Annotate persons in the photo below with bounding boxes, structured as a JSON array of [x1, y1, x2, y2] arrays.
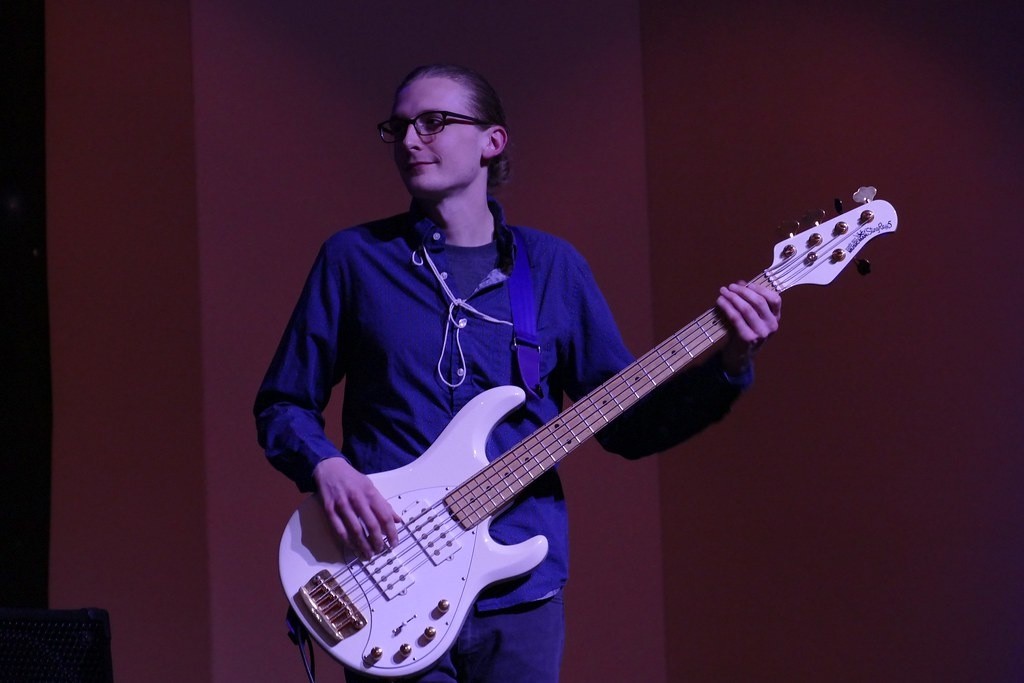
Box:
[[250, 62, 788, 683]]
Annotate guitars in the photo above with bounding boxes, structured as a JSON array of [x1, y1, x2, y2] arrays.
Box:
[[278, 186, 899, 676]]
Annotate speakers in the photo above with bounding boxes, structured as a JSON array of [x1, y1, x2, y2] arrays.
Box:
[[0, 608, 115, 683]]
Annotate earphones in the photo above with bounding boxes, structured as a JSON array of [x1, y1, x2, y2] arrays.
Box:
[[459, 318, 467, 328]]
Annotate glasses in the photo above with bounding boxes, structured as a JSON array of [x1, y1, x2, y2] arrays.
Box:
[[376, 110, 497, 143]]
